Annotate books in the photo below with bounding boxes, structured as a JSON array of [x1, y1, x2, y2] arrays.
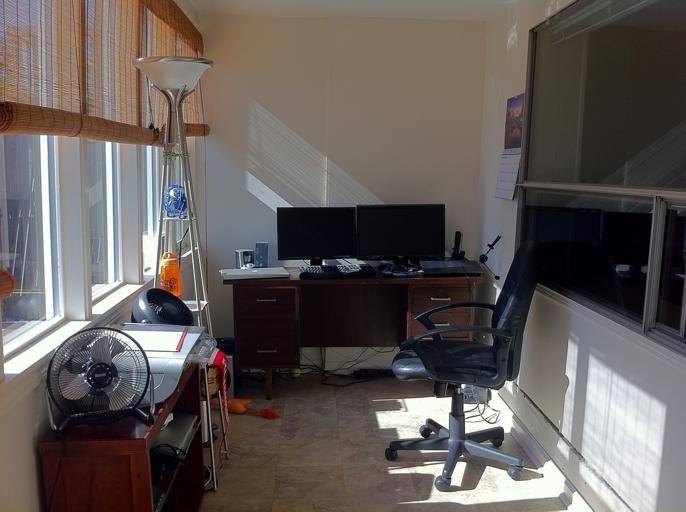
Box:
[[421, 257, 483, 277], [220, 266, 290, 280]]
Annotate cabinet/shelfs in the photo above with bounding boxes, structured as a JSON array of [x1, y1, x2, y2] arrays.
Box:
[[129, 55, 213, 335], [232, 283, 301, 400], [40, 365, 203, 511], [398, 286, 475, 346]]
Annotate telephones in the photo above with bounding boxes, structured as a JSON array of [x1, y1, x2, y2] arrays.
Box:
[[451, 230, 465, 260]]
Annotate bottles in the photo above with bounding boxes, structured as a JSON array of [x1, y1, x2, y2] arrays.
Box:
[[160, 252, 183, 302]]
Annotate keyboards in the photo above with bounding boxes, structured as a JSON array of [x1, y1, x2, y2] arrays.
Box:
[[296, 265, 376, 279]]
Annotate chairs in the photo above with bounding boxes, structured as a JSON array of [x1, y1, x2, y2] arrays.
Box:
[[384, 232, 550, 491]]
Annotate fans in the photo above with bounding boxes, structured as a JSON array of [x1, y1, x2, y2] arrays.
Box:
[[46, 326, 156, 434]]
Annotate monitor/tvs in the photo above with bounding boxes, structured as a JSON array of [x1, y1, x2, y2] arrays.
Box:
[[356, 203, 445, 271], [277, 207, 355, 267]]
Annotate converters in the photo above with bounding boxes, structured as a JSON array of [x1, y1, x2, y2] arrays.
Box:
[[357, 369, 378, 378], [151, 450, 176, 476]]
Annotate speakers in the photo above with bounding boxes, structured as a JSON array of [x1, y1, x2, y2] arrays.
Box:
[[256, 242, 268, 267]]
